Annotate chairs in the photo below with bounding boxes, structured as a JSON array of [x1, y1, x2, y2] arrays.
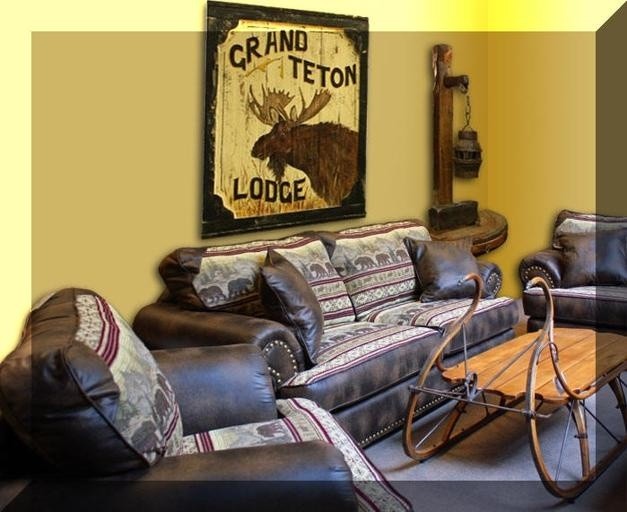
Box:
[[518, 211, 627, 333], [0, 287, 411, 511]]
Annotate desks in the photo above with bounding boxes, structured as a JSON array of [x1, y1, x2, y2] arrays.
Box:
[[403, 272, 626, 500]]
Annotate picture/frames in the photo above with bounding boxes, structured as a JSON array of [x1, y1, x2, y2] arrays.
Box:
[[201, 0, 369, 239]]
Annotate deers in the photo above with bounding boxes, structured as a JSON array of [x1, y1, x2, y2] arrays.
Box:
[[247, 84, 358, 208]]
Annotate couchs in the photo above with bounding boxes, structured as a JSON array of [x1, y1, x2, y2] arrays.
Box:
[[132, 287, 519, 450]]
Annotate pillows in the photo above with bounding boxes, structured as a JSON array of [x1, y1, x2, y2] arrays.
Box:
[[159, 233, 356, 324], [259, 245, 324, 366], [405, 238, 491, 301], [554, 218, 627, 289], [320, 220, 431, 318]]
